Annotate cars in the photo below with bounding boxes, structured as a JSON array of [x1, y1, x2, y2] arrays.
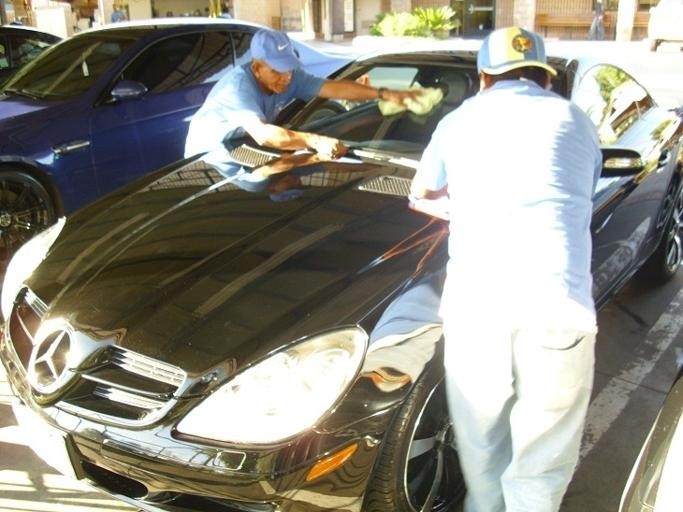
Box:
[[648, 0, 683, 52]]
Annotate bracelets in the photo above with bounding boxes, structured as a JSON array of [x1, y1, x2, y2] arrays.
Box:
[[378, 88, 388, 99]]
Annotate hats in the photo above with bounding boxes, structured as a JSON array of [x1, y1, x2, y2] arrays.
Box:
[[476, 24, 559, 78], [249, 28, 308, 74]]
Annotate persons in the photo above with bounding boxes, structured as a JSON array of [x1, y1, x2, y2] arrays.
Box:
[[181, 28, 423, 159], [408, 27, 601, 510], [110, 3, 126, 25], [197, 144, 324, 202], [167, 2, 234, 22], [582, 1, 606, 41]]
